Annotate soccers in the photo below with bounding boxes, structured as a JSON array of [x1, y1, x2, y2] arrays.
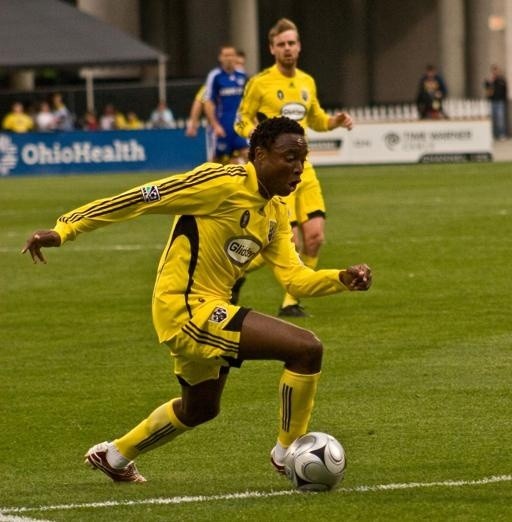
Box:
[[285, 431, 346, 490]]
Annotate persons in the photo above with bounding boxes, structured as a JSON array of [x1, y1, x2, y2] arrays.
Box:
[[181, 48, 250, 164], [483, 62, 510, 141], [21, 113, 373, 481], [230, 17, 354, 318], [416, 63, 452, 118], [2, 92, 177, 133]]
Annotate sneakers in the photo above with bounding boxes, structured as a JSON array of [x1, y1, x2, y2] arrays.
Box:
[[270, 446, 286, 475], [277, 301, 308, 317], [84, 440, 148, 483]]
